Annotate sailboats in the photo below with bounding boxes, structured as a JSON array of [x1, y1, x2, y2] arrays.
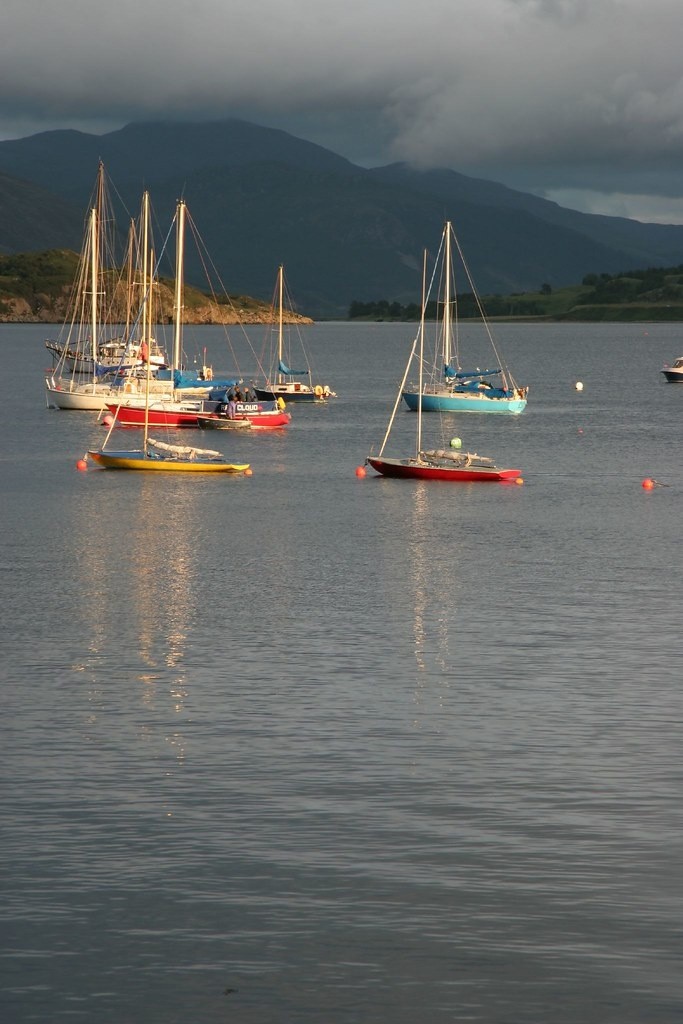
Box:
[[86, 250, 249, 473], [400, 221, 529, 415], [44, 159, 292, 429], [250, 267, 323, 400], [366, 248, 521, 481]]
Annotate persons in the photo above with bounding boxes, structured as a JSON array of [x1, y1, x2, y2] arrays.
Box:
[[226, 397, 238, 420], [243, 386, 258, 402], [234, 387, 246, 403]]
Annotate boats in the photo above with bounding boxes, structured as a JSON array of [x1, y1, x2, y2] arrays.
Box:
[[661, 357, 683, 383]]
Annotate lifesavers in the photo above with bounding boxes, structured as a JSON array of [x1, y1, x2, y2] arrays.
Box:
[[315, 385, 323, 396], [278, 397, 286, 409]]
